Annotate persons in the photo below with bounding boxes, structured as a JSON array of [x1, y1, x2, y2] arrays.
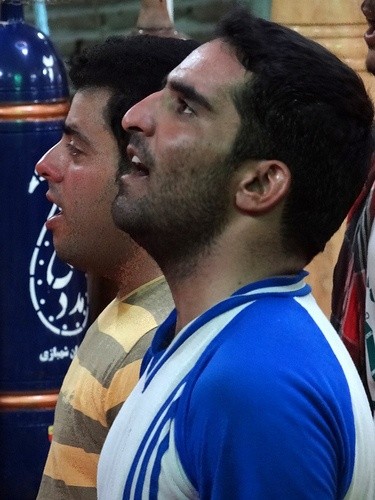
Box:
[[329, 1, 375, 425], [35, 36, 212, 500], [95, 10, 374, 500]]
